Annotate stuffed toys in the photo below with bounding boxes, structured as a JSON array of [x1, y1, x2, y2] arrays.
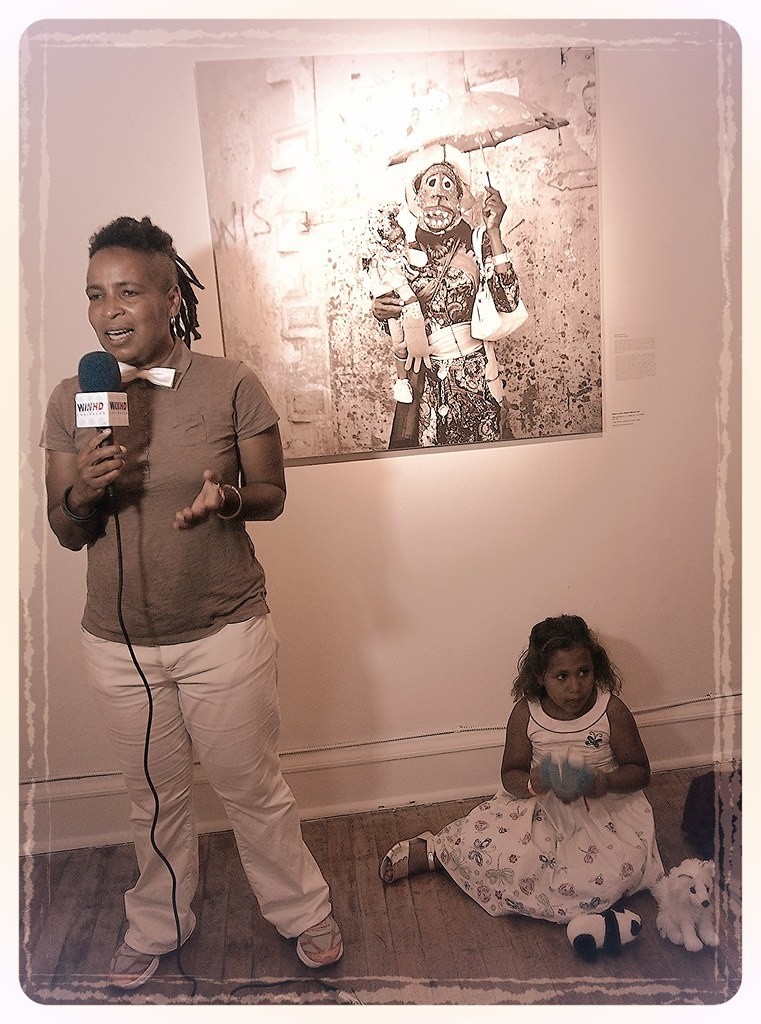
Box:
[[653, 858, 720, 952], [566, 906, 643, 962]]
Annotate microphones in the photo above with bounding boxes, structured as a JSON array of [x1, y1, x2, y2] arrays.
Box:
[[74, 350, 129, 499]]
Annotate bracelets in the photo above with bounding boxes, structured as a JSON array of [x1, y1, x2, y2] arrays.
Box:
[[60, 485, 97, 522], [492, 252, 511, 266], [211, 482, 243, 520], [528, 778, 540, 797]]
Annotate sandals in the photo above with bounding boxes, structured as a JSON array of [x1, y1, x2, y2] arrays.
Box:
[[378, 830, 436, 884]]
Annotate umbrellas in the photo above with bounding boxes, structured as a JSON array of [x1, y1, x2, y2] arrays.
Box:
[[385, 90, 570, 185]]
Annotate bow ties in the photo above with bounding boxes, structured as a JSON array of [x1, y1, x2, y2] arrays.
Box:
[[117, 361, 175, 388]]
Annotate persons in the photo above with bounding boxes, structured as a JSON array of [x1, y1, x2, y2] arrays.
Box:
[[368, 162, 528, 450], [40, 215, 344, 990], [379, 613, 664, 953]]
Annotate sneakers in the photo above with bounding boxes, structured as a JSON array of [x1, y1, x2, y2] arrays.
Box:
[[296, 914, 343, 968], [109, 942, 159, 988]]
